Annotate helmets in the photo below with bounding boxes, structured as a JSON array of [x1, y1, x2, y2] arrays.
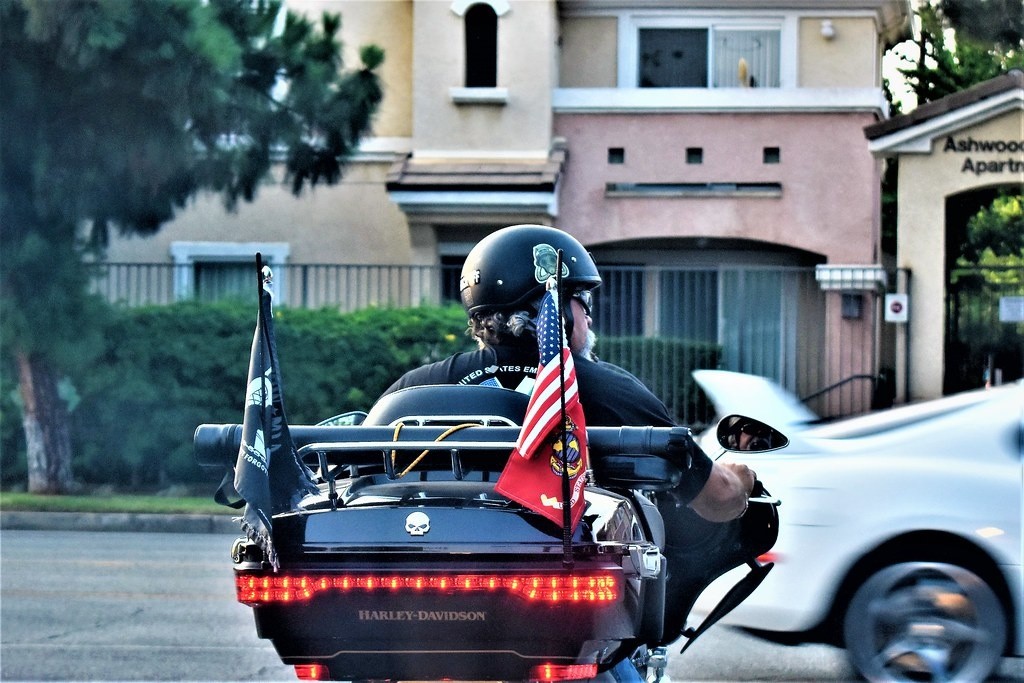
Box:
[[729, 416, 743, 433], [457, 223, 603, 325]]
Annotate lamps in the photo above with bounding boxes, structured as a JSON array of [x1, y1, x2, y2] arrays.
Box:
[[820, 21, 835, 40]]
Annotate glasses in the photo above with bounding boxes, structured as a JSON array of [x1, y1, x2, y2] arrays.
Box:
[[569, 287, 594, 318], [739, 422, 774, 435]]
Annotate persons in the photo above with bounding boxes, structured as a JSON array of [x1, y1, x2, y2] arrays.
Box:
[[358, 222, 756, 683], [727, 415, 772, 450]]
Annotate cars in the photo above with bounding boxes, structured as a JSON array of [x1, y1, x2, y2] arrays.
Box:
[[676, 368, 1024, 682]]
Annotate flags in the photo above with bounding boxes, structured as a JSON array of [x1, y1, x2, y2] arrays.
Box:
[[494, 275, 593, 536], [232, 285, 320, 572]]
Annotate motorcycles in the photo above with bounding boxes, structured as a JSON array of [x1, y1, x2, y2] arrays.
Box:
[[195, 248, 792, 683]]
